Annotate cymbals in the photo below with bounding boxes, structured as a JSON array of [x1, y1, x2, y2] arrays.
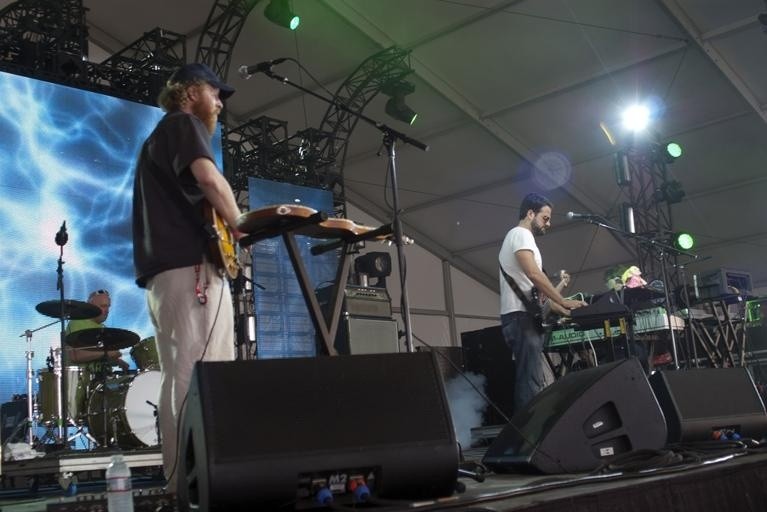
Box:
[[36, 299, 101, 320], [65, 328, 140, 352]]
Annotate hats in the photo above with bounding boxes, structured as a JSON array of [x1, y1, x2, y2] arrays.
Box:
[[167, 63, 234, 100]]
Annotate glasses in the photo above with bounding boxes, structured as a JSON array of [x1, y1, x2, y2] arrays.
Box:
[[89, 289, 109, 301]]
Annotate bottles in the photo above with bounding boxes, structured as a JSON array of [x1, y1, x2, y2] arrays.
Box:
[[105, 454, 133, 511], [67, 433, 75, 449]]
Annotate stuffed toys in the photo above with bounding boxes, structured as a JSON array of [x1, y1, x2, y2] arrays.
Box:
[[619, 265, 647, 289]]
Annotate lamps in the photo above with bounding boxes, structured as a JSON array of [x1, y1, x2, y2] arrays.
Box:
[[383, 92, 417, 126], [262, 1, 300, 32]]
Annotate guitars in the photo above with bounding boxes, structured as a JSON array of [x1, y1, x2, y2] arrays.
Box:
[[203, 198, 243, 279], [532, 269, 571, 336], [236, 203, 414, 245]]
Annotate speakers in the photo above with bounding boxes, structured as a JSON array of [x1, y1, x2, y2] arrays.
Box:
[[0, 400, 35, 445], [416, 346, 470, 385], [461, 324, 515, 427], [176, 351, 460, 512], [647, 367, 767, 445], [481, 356, 668, 477]]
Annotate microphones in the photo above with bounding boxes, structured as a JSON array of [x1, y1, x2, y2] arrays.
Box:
[[238, 57, 283, 80], [567, 212, 600, 221], [55, 221, 68, 245]]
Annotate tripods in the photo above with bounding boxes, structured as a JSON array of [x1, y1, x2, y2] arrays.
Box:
[[32, 270, 89, 455], [32, 365, 100, 449], [4, 334, 58, 446]]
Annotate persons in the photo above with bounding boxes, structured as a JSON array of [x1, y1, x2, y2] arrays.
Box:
[[62, 289, 131, 419], [541, 268, 572, 318], [495, 191, 591, 416], [132, 62, 252, 498]]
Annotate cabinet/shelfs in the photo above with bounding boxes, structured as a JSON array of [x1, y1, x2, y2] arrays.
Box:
[[231, 203, 401, 357], [686, 290, 759, 369]]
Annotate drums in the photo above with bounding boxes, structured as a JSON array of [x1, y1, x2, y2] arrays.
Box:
[[130, 336, 161, 374], [88, 368, 161, 451], [36, 365, 93, 428]]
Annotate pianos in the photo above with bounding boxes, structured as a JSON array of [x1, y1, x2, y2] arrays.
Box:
[[571, 287, 633, 331]]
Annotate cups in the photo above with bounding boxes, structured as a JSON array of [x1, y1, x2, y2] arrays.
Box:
[[745, 300, 760, 322]]
